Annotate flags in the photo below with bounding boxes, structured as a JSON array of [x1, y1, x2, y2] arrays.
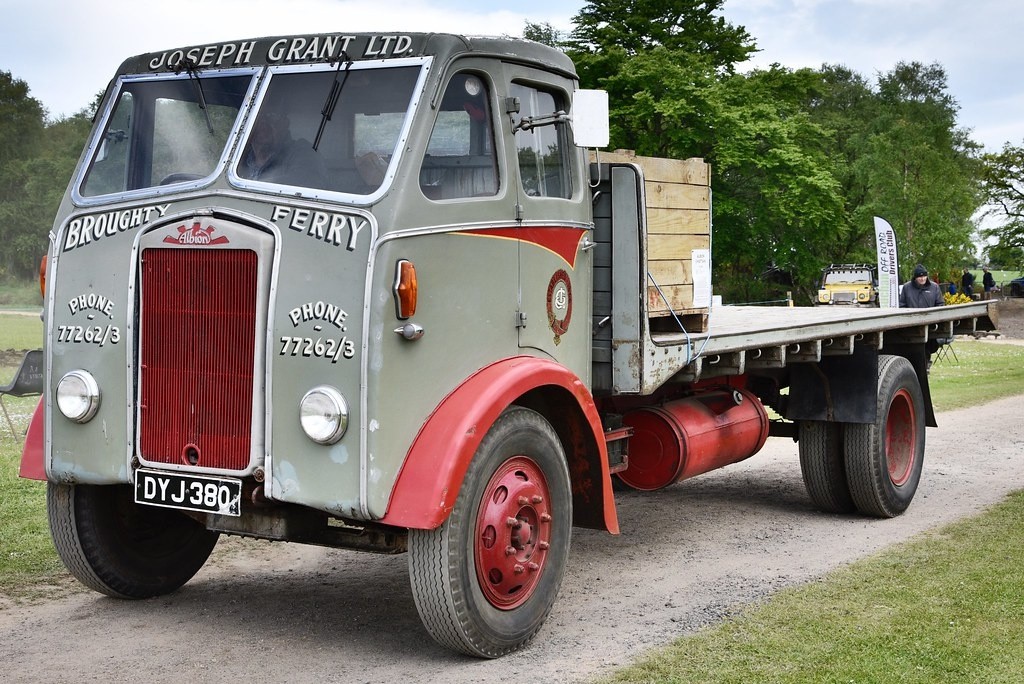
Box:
[[872, 215, 900, 310]]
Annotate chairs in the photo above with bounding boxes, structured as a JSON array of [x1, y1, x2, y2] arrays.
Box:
[[0, 349, 44, 446], [933, 337, 960, 366]]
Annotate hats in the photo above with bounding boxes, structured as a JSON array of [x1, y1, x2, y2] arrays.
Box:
[[914, 264, 928, 280]]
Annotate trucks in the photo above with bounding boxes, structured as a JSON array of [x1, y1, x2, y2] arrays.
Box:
[[814, 262, 880, 308], [16, 31, 1002, 665]]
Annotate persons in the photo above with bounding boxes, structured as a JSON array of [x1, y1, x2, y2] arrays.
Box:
[[982, 265, 994, 292], [898, 263, 947, 307], [950, 281, 957, 294], [238, 94, 328, 195], [960, 266, 976, 299]]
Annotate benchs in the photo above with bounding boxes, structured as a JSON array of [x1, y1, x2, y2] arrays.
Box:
[[323, 155, 499, 199]]
